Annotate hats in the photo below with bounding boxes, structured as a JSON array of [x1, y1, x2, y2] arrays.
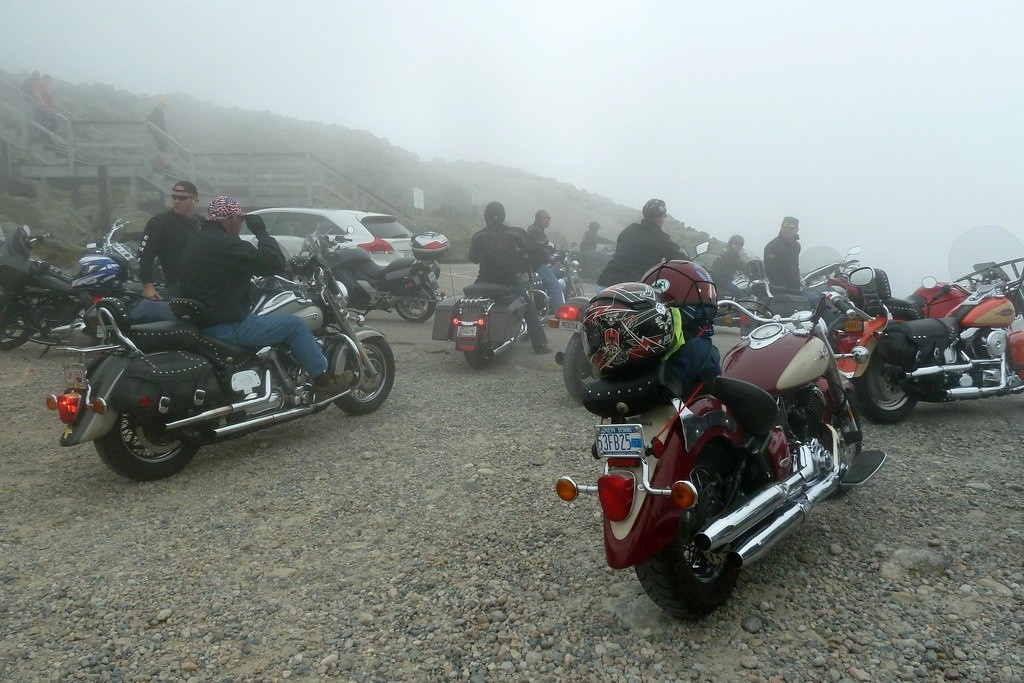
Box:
[[173, 181, 198, 196], [205, 196, 246, 221], [643, 199, 666, 217]]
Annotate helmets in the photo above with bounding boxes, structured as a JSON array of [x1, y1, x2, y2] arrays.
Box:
[[641, 260, 717, 322], [582, 282, 674, 376]]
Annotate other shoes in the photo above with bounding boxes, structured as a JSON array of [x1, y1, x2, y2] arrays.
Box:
[[535, 347, 553, 354]]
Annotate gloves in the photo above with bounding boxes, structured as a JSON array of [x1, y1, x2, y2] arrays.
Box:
[[244, 215, 266, 234]]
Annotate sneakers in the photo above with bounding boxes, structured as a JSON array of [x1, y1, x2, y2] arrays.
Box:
[[313, 371, 353, 396]]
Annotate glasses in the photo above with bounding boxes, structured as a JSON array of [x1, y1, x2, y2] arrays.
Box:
[[172, 195, 192, 201], [781, 225, 798, 231]]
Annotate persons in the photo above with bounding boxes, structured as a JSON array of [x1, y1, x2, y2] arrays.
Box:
[[528, 210, 563, 315], [180, 196, 353, 401], [712, 235, 746, 299], [764, 216, 801, 296], [137, 181, 206, 300], [597, 199, 693, 295], [580, 222, 614, 262], [468, 201, 553, 355]]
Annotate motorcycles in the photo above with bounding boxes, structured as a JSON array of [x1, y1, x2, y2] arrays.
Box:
[[529, 244, 587, 325], [291, 219, 450, 323], [430, 251, 550, 370], [78, 216, 167, 284], [0, 217, 127, 350], [554, 249, 888, 622], [47, 220, 393, 481], [690, 243, 987, 399], [836, 258, 1023, 424]]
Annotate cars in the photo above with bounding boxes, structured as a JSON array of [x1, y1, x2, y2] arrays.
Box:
[[236, 207, 414, 277]]
